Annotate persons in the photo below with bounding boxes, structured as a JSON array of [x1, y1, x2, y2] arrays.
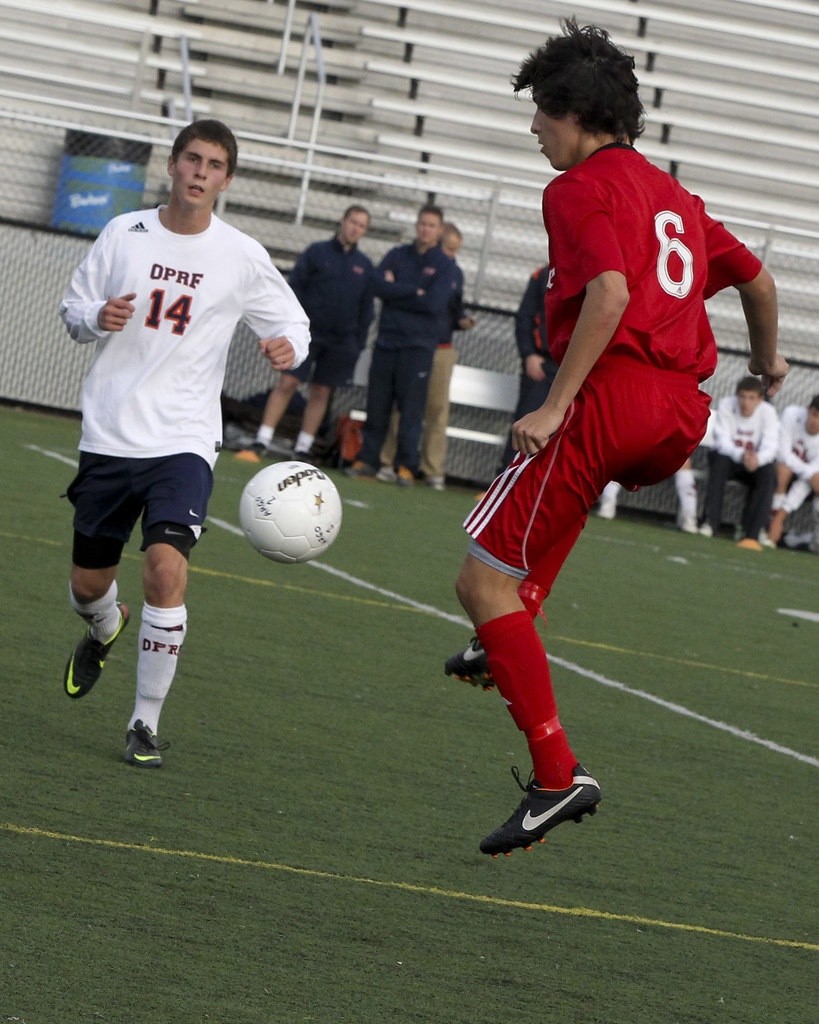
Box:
[[235, 202, 478, 492], [53, 120, 315, 766], [592, 370, 819, 552], [430, 21, 792, 861]]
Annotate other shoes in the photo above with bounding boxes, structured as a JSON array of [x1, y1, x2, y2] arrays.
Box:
[[599, 496, 617, 520], [350, 460, 377, 479], [236, 442, 266, 464], [699, 521, 714, 537], [425, 475, 446, 491], [396, 464, 415, 486], [734, 524, 747, 544], [756, 526, 778, 550], [680, 516, 699, 534], [290, 447, 313, 466], [375, 467, 398, 483]]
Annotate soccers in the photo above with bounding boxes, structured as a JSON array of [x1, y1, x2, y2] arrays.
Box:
[[237, 459, 345, 566]]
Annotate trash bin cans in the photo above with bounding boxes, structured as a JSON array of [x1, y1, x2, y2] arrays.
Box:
[[47, 125, 156, 243]]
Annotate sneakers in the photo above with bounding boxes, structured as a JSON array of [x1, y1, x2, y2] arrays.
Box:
[[123, 719, 172, 768], [445, 636, 496, 691], [63, 601, 130, 698], [479, 762, 601, 858]]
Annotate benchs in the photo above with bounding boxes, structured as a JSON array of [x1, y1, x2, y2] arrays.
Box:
[[0, 1, 817, 364], [348, 348, 519, 445]]
